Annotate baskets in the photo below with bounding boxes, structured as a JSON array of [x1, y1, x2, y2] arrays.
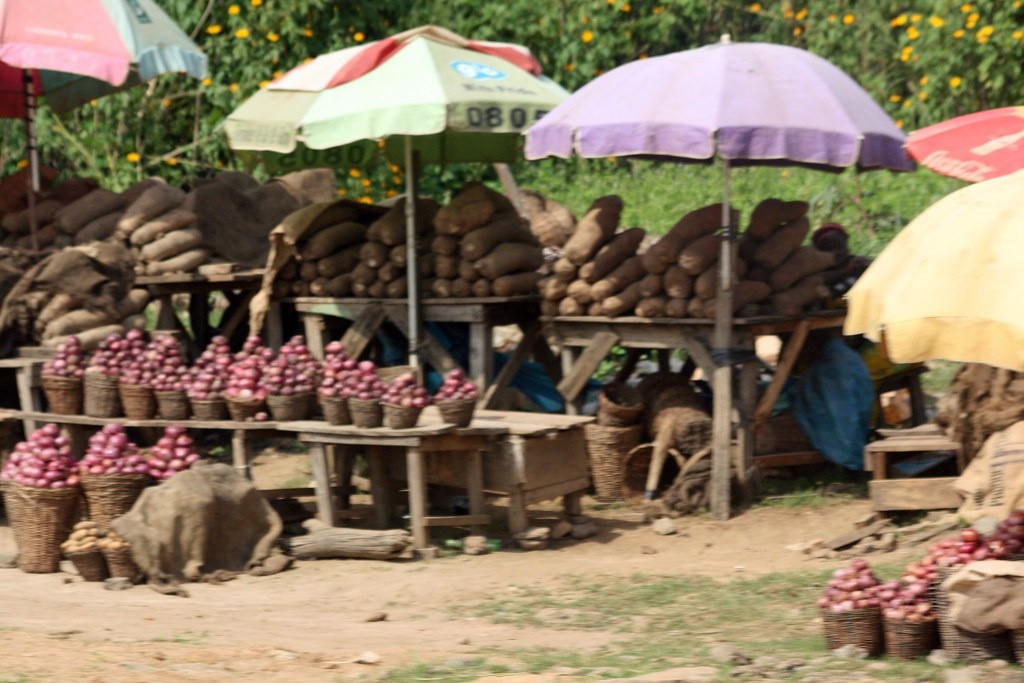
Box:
[[96, 544, 142, 579], [435, 397, 478, 428], [2, 480, 80, 573], [1011, 626, 1024, 668], [582, 423, 644, 502], [62, 545, 111, 581], [78, 473, 149, 528], [817, 602, 884, 658], [595, 390, 645, 429], [38, 374, 428, 429], [880, 611, 939, 661], [645, 383, 715, 455], [933, 565, 1012, 663]]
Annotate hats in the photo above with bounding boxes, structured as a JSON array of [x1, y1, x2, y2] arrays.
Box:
[[811, 222, 850, 247]]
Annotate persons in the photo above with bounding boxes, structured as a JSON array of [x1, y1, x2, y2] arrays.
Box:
[[778, 215, 870, 375]]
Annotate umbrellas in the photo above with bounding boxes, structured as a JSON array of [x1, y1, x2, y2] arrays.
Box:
[[519, 32, 917, 520], [900, 98, 1024, 191], [2, 0, 211, 258], [842, 163, 1024, 399], [222, 24, 578, 410]]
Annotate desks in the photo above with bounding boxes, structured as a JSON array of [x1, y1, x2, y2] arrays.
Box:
[[0, 264, 975, 546]]
